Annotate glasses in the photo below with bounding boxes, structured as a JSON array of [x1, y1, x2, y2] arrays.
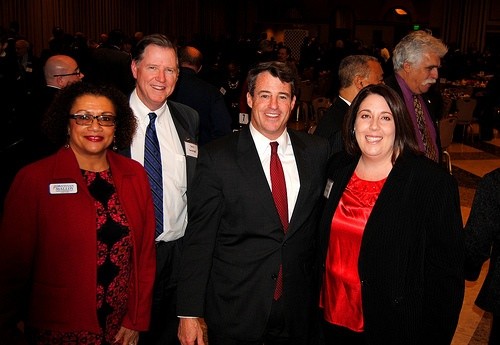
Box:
[[68, 113, 116, 126], [54, 69, 80, 77]]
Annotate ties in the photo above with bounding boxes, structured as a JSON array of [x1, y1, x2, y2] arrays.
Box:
[[270, 141, 288, 302], [144, 113, 163, 239], [413, 95, 437, 162]]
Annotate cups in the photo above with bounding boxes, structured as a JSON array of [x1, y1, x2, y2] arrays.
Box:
[[294, 103, 302, 122]]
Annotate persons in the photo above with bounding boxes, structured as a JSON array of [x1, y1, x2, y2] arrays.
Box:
[[0, 1, 500, 345]]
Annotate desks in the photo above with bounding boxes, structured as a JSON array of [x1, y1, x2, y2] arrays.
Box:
[[441, 88, 484, 142]]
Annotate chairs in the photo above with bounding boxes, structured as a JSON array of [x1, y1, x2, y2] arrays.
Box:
[[452, 97, 477, 144], [436, 116, 454, 174], [296, 80, 315, 122], [312, 97, 332, 134], [442, 98, 452, 119]]
